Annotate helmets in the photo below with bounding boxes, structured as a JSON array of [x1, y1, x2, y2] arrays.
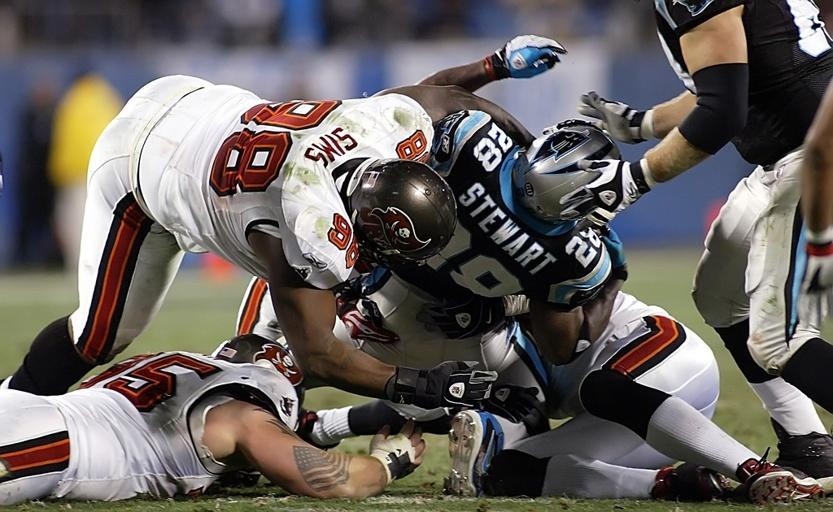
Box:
[[510, 118, 625, 227], [207, 333, 307, 412], [348, 157, 459, 269]]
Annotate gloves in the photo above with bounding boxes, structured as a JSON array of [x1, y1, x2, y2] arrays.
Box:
[[392, 360, 500, 412], [798, 225, 833, 299], [559, 157, 655, 231], [576, 89, 656, 146], [492, 33, 569, 83]]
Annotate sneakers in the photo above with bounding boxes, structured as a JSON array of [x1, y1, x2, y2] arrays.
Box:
[[649, 462, 737, 504], [447, 409, 506, 499], [736, 417, 832, 508]]
